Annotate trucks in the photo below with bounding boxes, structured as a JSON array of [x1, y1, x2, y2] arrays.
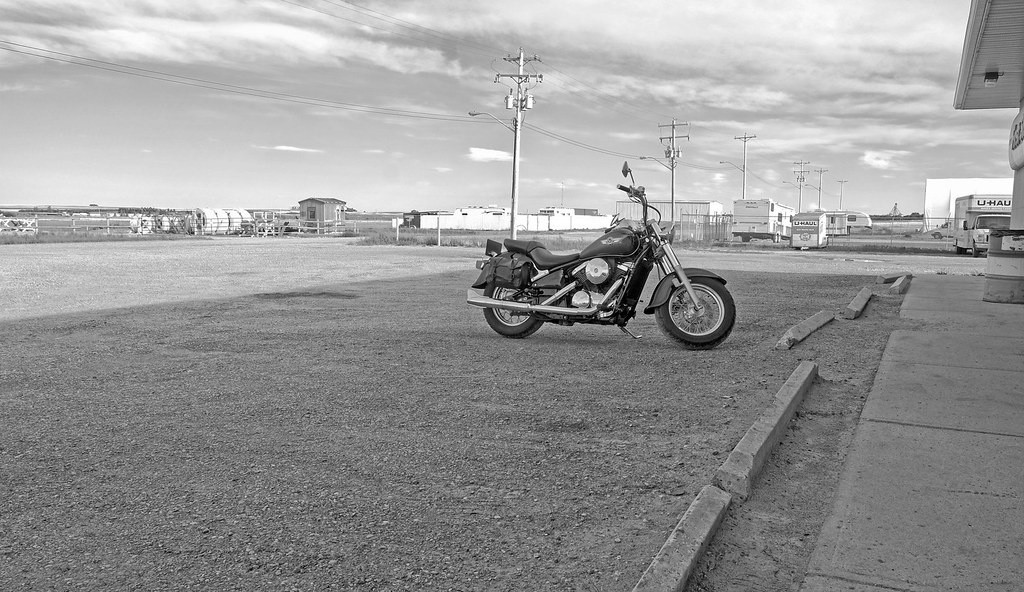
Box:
[[731, 198, 795, 243], [953, 193, 1013, 258]]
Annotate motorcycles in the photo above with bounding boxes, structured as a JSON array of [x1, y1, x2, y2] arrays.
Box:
[[465, 160, 737, 350]]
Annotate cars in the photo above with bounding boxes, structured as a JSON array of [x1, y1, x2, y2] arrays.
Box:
[[931, 221, 954, 240]]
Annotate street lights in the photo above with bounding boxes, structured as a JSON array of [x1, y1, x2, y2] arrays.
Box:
[[782, 181, 802, 213], [468, 109, 521, 243], [719, 160, 747, 199], [805, 183, 822, 209], [639, 155, 675, 228]]
